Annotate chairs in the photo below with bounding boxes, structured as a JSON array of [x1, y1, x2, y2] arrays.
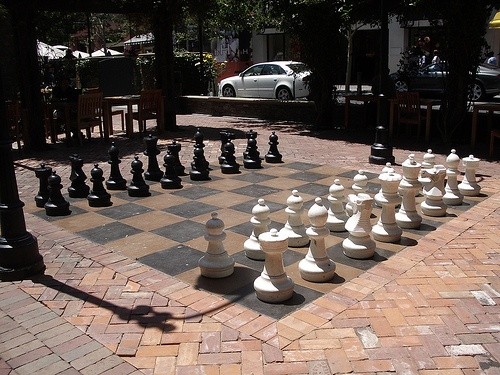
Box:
[[7, 88, 164, 155], [396, 91, 427, 140], [490, 129, 500, 160]]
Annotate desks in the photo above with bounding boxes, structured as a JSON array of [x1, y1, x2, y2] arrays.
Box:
[[342, 93, 387, 132], [46, 101, 74, 145], [102, 95, 142, 137], [386, 97, 441, 140], [471, 101, 500, 148]]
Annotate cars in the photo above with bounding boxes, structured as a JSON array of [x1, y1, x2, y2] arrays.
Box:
[[216, 61, 313, 100], [388, 61, 500, 102]]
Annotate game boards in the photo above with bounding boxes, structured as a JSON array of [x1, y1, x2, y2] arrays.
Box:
[[23, 155, 495, 321]]
